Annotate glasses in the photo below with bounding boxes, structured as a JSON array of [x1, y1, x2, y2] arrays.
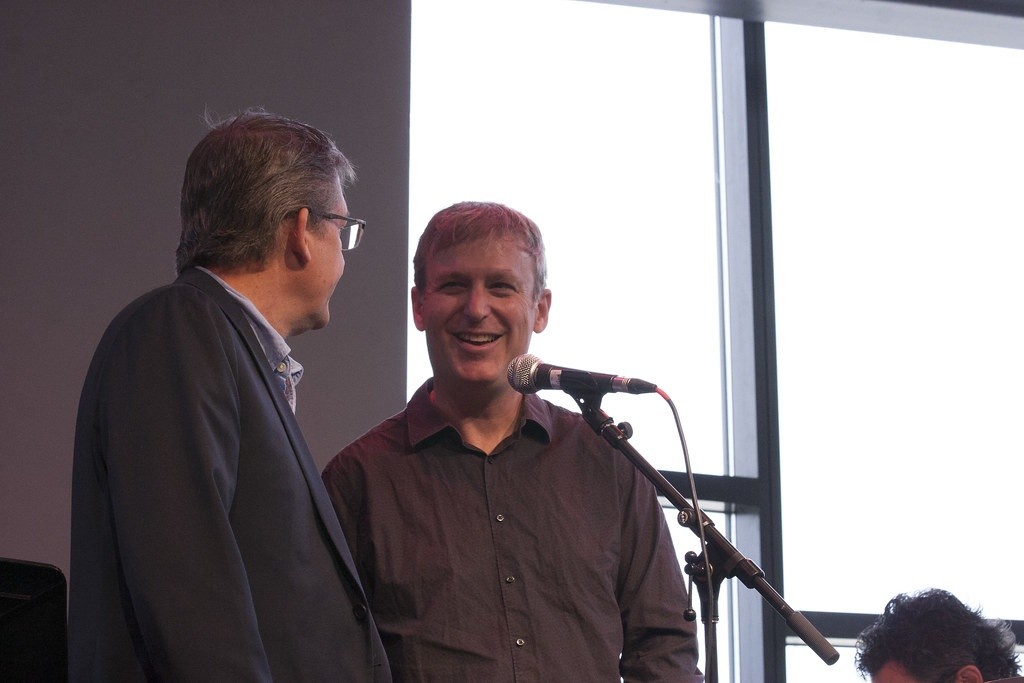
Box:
[[311, 210, 366, 252]]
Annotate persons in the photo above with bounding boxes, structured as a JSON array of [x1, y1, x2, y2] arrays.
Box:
[[67, 110, 396, 683], [854, 588, 1024, 683], [322, 201, 705, 683]]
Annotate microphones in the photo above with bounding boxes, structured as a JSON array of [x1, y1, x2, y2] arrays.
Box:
[[507, 353, 657, 394]]
